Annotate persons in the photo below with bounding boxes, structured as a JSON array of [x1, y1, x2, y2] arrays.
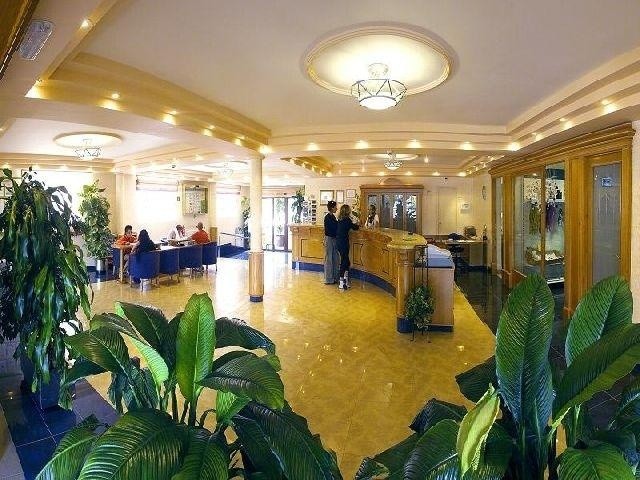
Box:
[[365, 204, 379, 229], [117, 224, 137, 246], [187, 222, 210, 244], [123, 229, 155, 284], [324, 201, 340, 286], [336, 204, 360, 289], [167, 224, 185, 246]]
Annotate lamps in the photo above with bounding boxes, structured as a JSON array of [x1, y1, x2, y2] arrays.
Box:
[[53, 131, 124, 164], [382, 149, 404, 172], [303, 24, 452, 112]]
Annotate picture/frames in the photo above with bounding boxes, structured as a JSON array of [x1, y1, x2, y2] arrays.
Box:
[[346, 189, 357, 198], [336, 190, 345, 204], [319, 190, 335, 205]]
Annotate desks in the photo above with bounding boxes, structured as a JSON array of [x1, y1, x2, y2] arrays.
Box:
[[442, 239, 485, 267], [110, 239, 195, 284]]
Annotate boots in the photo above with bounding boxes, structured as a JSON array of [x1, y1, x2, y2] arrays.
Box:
[[339, 280, 348, 290], [344, 276, 351, 288]]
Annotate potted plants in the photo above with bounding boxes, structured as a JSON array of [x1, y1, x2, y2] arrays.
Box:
[[404, 282, 436, 331], [32, 292, 344, 480], [240, 196, 251, 250], [353, 268, 639, 479], [0, 164, 95, 412], [290, 188, 305, 224], [78, 178, 120, 283], [275, 198, 285, 248]]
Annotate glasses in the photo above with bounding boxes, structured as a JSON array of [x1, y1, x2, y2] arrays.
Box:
[[367, 208, 375, 212]]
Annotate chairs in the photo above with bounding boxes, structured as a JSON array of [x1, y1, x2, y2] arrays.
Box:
[[447, 233, 466, 266], [180, 244, 205, 279], [127, 250, 160, 292], [203, 241, 218, 271], [160, 248, 181, 287], [112, 247, 120, 277]]
